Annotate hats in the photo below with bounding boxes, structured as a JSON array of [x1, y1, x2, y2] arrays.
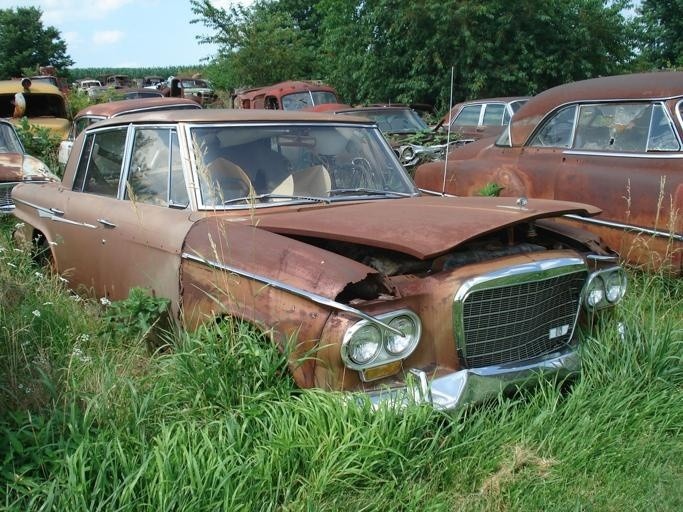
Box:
[[7, 97, 633, 422], [412, 70, 682, 282]]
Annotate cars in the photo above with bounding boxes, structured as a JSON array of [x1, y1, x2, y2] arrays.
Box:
[[57, 97, 202, 179], [318, 104, 476, 180], [1, 73, 218, 107], [0, 78, 72, 145], [412, 70, 682, 282], [230, 80, 340, 147], [1, 119, 62, 221], [429, 96, 528, 143], [7, 97, 633, 422]]
